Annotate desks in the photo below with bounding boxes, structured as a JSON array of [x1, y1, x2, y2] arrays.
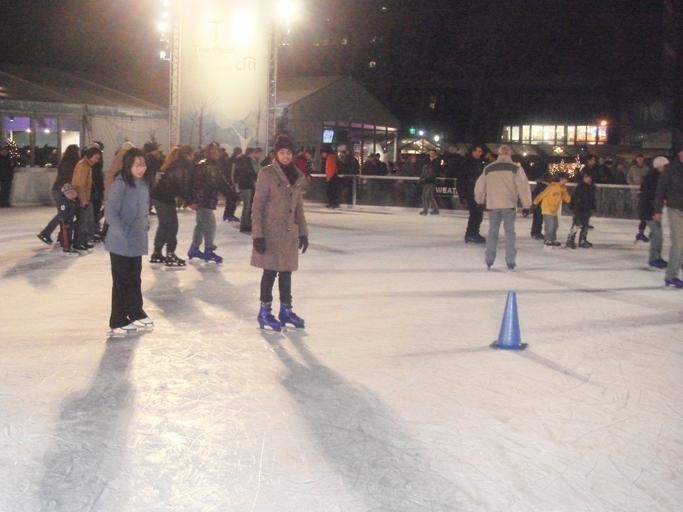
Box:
[[595, 118, 608, 156]]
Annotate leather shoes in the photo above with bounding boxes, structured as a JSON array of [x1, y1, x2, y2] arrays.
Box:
[[253, 238, 266, 253], [298, 236, 308, 253]]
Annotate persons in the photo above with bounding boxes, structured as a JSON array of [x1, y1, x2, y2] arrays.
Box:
[[38, 142, 105, 255], [627, 150, 682, 288], [105, 147, 154, 333], [249, 134, 309, 335], [474, 145, 532, 270], [149, 142, 231, 268], [318, 148, 459, 216], [456, 146, 487, 243], [223, 146, 262, 231], [46, 148, 57, 166], [2, 147, 14, 207], [531, 155, 652, 249], [144, 141, 163, 215]]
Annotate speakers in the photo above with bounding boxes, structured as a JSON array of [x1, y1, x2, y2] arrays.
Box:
[[151, 250, 185, 264], [189, 244, 222, 263], [257, 302, 305, 328]]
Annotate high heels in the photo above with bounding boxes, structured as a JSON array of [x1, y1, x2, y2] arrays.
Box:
[[489, 290, 529, 350]]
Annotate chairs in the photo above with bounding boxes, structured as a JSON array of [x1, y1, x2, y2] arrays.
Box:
[[62, 243, 94, 254], [113, 316, 154, 331], [532, 232, 591, 248], [465, 233, 485, 242], [40, 231, 54, 242], [650, 257, 668, 267], [636, 234, 649, 241], [665, 277, 683, 287]]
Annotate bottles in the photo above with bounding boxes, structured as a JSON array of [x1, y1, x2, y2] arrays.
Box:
[[653, 156, 670, 168], [275, 135, 294, 154]]
